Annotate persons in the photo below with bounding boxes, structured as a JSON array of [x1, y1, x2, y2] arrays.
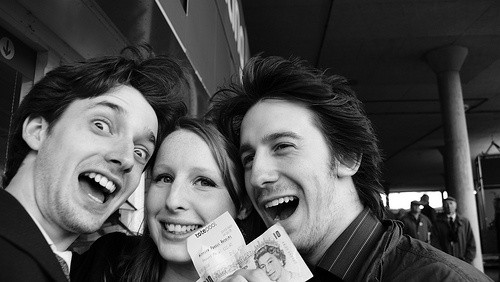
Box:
[[208, 53, 495, 282], [69, 116, 253, 282], [0, 43, 190, 282], [400, 200, 432, 243], [430, 196, 476, 263], [420, 194, 436, 224]]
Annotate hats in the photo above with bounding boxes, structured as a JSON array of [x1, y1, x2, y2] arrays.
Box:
[[411, 201, 423, 206], [444, 197, 456, 201]]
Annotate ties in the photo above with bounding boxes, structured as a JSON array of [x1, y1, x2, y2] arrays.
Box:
[[450, 218, 454, 235]]
